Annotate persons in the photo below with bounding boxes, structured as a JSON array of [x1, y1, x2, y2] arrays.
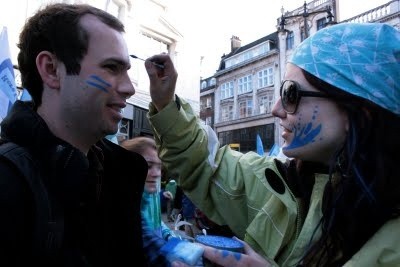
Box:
[[0, 3, 148, 267], [144, 21, 400, 267], [117, 135, 192, 267], [164, 175, 238, 241]]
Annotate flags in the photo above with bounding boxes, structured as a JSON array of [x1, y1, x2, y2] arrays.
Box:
[[0, 24, 20, 125]]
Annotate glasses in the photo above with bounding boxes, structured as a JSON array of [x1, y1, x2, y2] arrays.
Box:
[[280, 80, 342, 114]]
